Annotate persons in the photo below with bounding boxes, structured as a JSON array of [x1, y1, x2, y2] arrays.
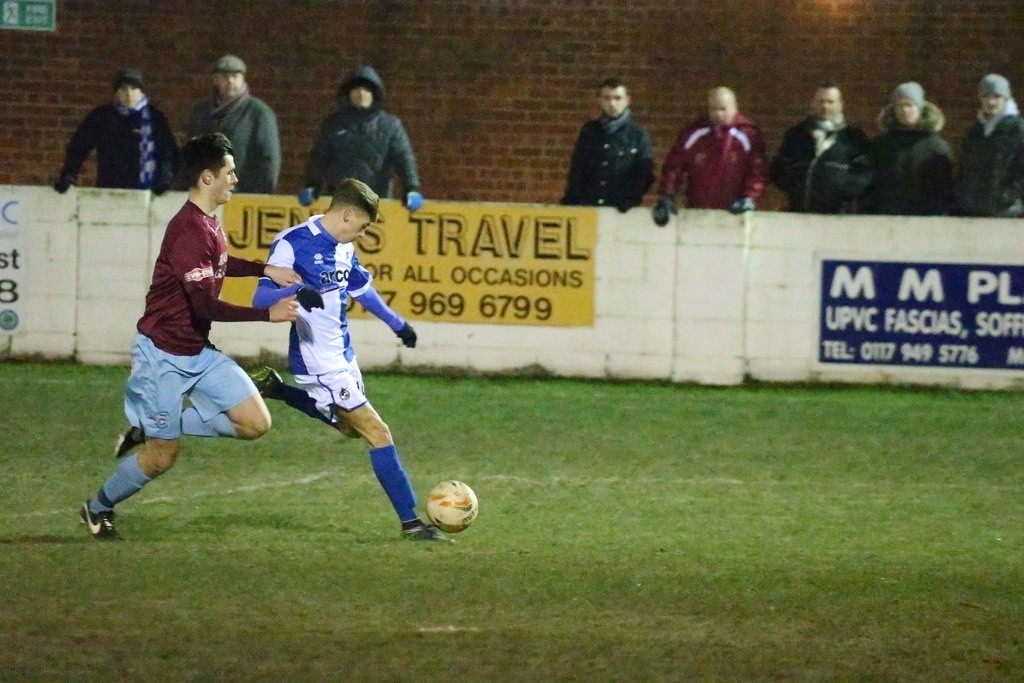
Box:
[[561, 79, 656, 213], [855, 81, 954, 215], [298, 64, 425, 212], [652, 87, 772, 226], [946, 72, 1024, 217], [53, 66, 180, 196], [770, 83, 876, 216], [189, 53, 280, 196], [78, 132, 304, 545], [249, 179, 449, 542]]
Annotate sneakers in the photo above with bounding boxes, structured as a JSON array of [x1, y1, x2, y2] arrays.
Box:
[[248, 366, 282, 398], [115, 425, 145, 456], [403, 520, 446, 541], [79, 499, 124, 542]]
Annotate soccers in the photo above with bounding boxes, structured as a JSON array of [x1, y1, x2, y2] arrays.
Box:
[[426, 480, 480, 534]]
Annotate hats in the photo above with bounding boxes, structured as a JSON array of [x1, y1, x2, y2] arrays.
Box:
[[980, 75, 1009, 97], [893, 81, 925, 110], [212, 55, 247, 73], [112, 69, 143, 90]]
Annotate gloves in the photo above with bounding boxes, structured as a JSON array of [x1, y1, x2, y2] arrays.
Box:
[[55, 175, 79, 194], [299, 186, 315, 206], [153, 178, 170, 194], [653, 196, 678, 225], [731, 196, 754, 214], [403, 191, 423, 211], [296, 286, 324, 313], [397, 323, 416, 349]]
